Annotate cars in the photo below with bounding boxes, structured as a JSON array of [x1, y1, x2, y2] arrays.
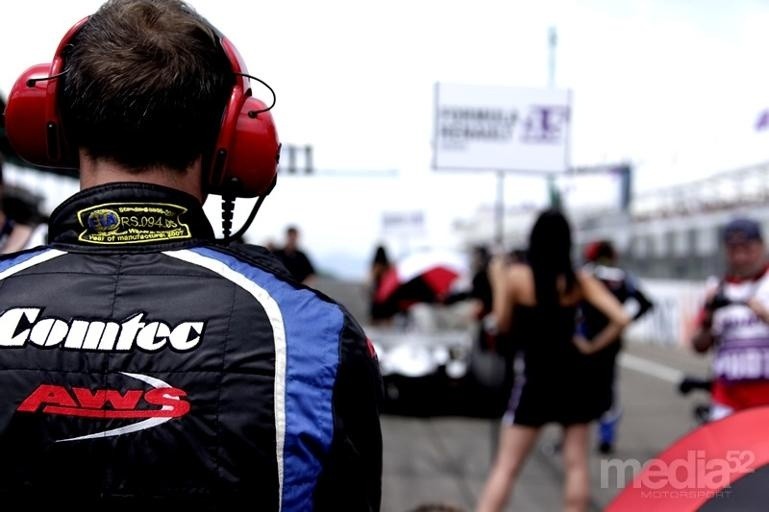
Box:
[[359, 305, 505, 415]]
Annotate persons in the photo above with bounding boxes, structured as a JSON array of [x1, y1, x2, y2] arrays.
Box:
[[365, 209, 653, 512], [269, 226, 318, 287], [690, 217, 769, 420], [0, 93, 49, 255], [0, 1, 380, 511]]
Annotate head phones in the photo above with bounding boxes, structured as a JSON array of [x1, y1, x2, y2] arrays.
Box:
[[0, 4, 282, 196]]
